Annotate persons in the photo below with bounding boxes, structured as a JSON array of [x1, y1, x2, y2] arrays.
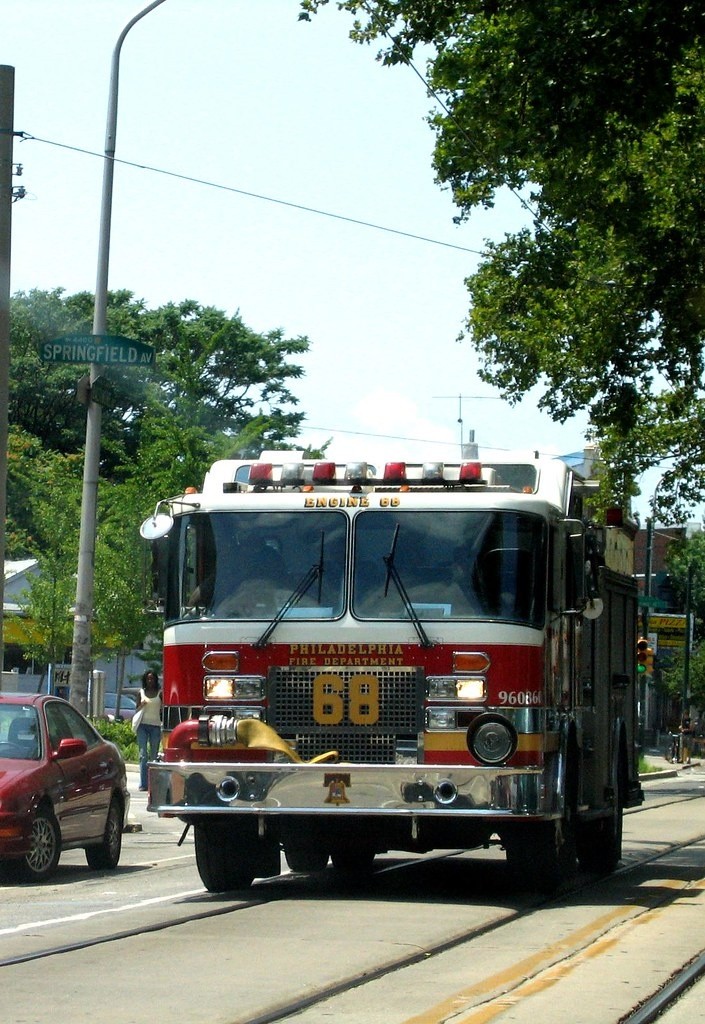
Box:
[[132, 670, 164, 793], [679, 709, 694, 764], [693, 710, 705, 757], [185, 532, 282, 607]]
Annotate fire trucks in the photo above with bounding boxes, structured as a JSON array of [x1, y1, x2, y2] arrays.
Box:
[[143, 449, 644, 899]]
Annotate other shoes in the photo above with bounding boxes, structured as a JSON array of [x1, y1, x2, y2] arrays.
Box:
[[139, 786, 148, 791]]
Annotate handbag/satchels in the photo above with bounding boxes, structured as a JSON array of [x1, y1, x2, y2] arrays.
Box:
[[132, 708, 144, 732]]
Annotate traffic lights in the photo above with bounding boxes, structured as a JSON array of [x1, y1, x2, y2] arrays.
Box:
[[644, 646, 656, 674], [637, 637, 648, 674]]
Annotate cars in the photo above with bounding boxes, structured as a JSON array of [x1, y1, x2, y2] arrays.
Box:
[[100, 693, 138, 723], [1, 691, 128, 881]]
